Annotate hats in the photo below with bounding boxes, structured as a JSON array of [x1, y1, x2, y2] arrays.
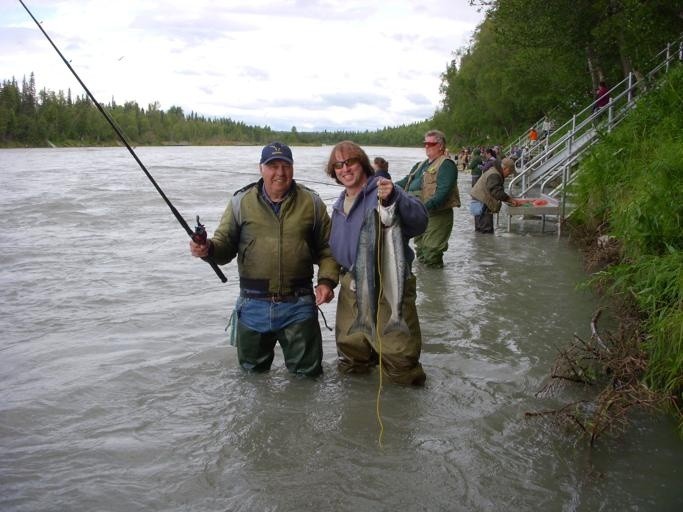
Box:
[[256, 142, 295, 167], [500, 156, 519, 176]]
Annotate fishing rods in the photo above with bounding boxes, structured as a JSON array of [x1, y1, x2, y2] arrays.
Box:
[[149, 165, 346, 202], [18, 0, 228, 283]]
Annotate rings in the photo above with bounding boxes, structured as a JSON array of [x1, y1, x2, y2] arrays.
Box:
[[379, 189, 383, 194]]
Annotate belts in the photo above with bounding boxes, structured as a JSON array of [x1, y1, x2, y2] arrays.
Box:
[[239, 287, 317, 305]]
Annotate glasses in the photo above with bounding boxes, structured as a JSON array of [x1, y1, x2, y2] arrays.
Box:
[[421, 141, 441, 149], [333, 156, 361, 169]]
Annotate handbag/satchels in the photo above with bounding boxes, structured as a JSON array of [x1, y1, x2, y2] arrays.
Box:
[[467, 199, 488, 217]]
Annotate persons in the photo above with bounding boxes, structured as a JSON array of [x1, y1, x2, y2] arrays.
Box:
[[324, 140, 429, 389], [453, 144, 528, 188], [469, 157, 519, 234], [392, 130, 461, 269], [528, 126, 537, 157], [541, 116, 554, 159], [370, 155, 390, 183], [189, 141, 339, 383], [590, 88, 602, 127], [594, 82, 610, 109]]
